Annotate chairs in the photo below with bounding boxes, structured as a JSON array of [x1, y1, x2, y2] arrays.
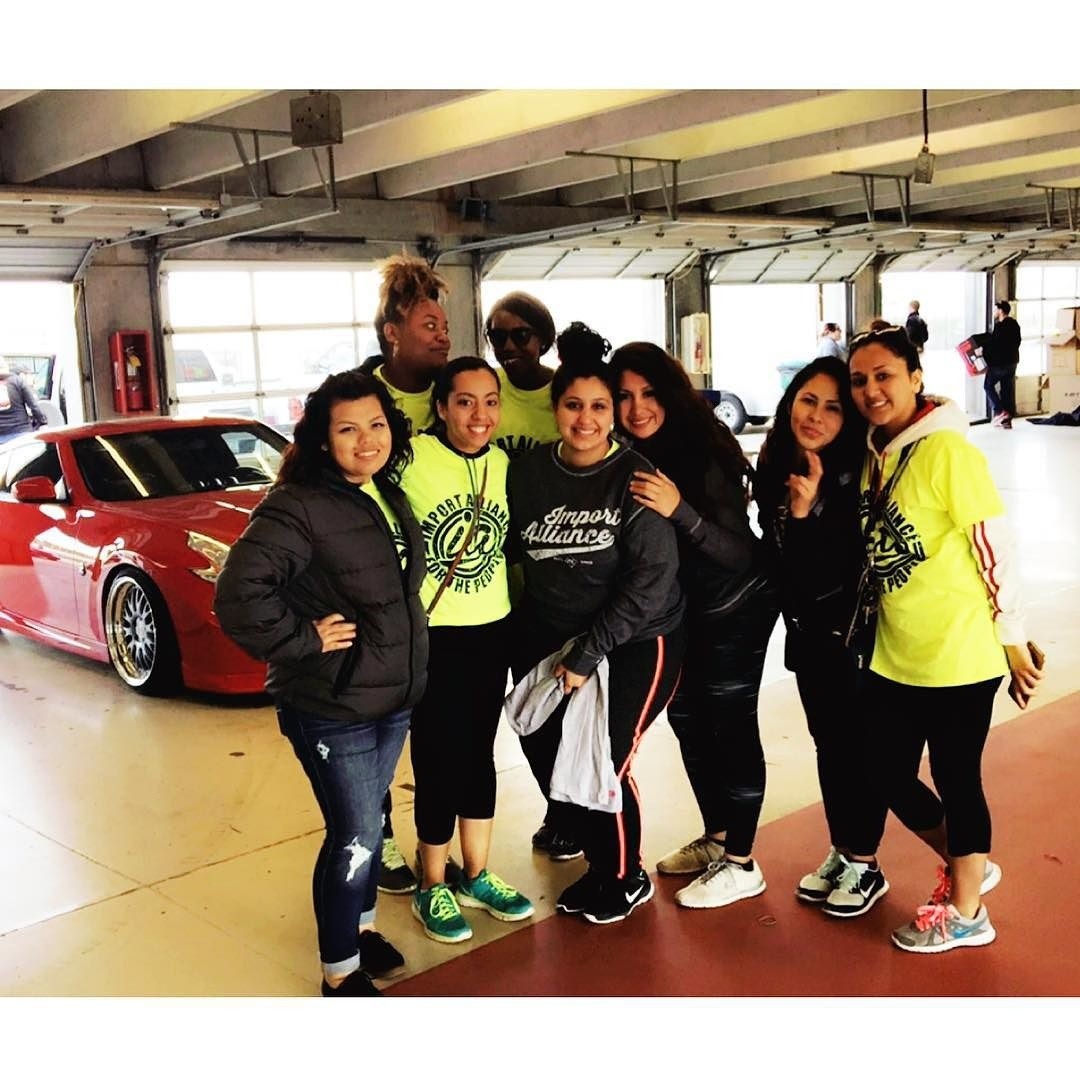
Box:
[[77, 446, 234, 497]]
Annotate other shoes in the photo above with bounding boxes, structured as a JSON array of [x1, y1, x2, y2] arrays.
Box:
[[1003, 420, 1012, 428], [549, 838, 584, 860], [992, 411, 1008, 426], [532, 823, 556, 847]]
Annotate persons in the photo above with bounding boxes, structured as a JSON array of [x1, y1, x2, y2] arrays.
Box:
[[906, 300, 929, 355], [844, 317, 1047, 956], [0, 357, 47, 444], [215, 255, 781, 997], [820, 322, 844, 359], [983, 301, 1021, 429]]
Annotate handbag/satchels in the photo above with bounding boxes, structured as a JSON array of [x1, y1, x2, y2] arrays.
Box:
[[846, 556, 881, 673]]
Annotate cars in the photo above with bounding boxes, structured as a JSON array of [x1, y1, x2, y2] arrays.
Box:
[[0, 341, 313, 483], [713, 358, 813, 435], [0, 414, 293, 698]]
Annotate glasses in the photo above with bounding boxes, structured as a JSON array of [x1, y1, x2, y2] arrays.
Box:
[[850, 326, 906, 346], [487, 326, 536, 349]]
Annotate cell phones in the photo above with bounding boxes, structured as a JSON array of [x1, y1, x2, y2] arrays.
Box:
[[1008, 640, 1045, 709]]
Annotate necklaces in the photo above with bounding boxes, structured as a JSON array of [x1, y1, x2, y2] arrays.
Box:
[[751, 353, 898, 919]]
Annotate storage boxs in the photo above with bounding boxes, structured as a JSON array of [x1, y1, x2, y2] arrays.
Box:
[[954, 304, 1080, 417]]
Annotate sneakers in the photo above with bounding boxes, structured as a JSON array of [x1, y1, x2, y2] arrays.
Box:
[[556, 870, 617, 912], [821, 855, 890, 917], [582, 869, 654, 924], [656, 837, 725, 873], [321, 970, 384, 996], [674, 855, 766, 908], [795, 846, 848, 900], [409, 878, 472, 943], [375, 838, 416, 892], [927, 853, 1002, 906], [413, 848, 464, 883], [356, 930, 405, 978], [455, 867, 533, 921], [892, 901, 996, 952]]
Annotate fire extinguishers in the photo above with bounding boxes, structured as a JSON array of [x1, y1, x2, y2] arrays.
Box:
[[696, 343, 703, 365], [123, 345, 144, 410]]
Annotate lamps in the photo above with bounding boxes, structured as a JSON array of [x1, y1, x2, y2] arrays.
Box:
[[909, 89, 938, 185]]
